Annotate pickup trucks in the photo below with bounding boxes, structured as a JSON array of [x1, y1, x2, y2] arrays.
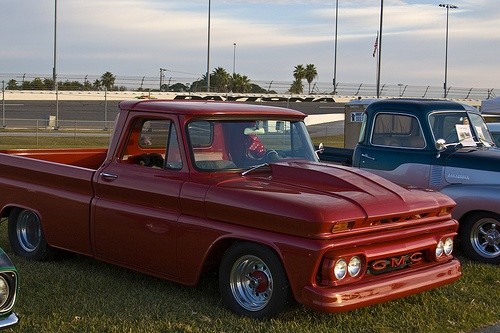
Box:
[[0, 99, 463, 320], [264, 98, 500, 264]]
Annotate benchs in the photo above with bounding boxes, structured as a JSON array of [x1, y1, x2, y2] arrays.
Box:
[[196, 159, 238, 170]]
